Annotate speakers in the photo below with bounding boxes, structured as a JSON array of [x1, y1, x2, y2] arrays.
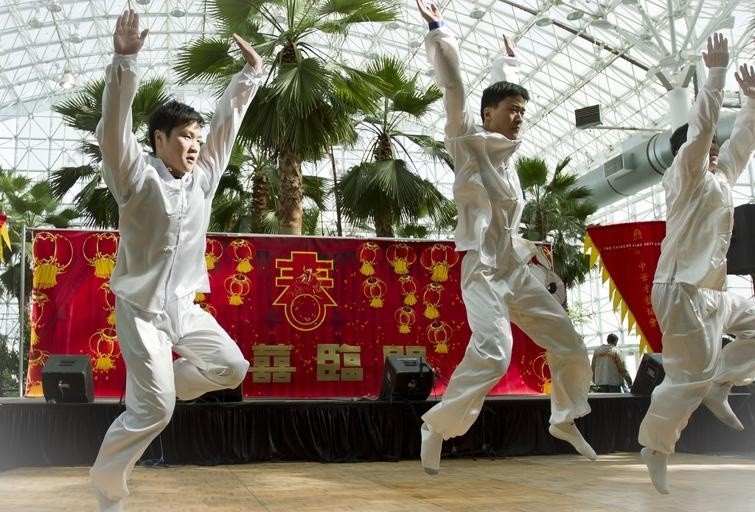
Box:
[[630, 353, 664, 395], [195, 383, 243, 403], [575, 104, 602, 129], [379, 356, 434, 401], [41, 354, 94, 403]]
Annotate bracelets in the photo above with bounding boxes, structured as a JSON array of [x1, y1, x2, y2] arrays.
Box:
[[428, 19, 445, 31], [508, 54, 515, 57]]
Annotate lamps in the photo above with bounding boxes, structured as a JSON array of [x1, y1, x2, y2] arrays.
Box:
[[467, 1, 615, 30]]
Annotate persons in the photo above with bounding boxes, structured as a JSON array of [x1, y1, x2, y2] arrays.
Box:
[[415, 0, 598, 476], [590, 333, 633, 393], [637, 32, 755, 494], [89, 8, 266, 511]]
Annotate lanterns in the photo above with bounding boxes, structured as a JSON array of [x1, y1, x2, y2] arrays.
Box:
[[359, 239, 463, 356], [192, 236, 255, 320], [23, 227, 121, 395]]
